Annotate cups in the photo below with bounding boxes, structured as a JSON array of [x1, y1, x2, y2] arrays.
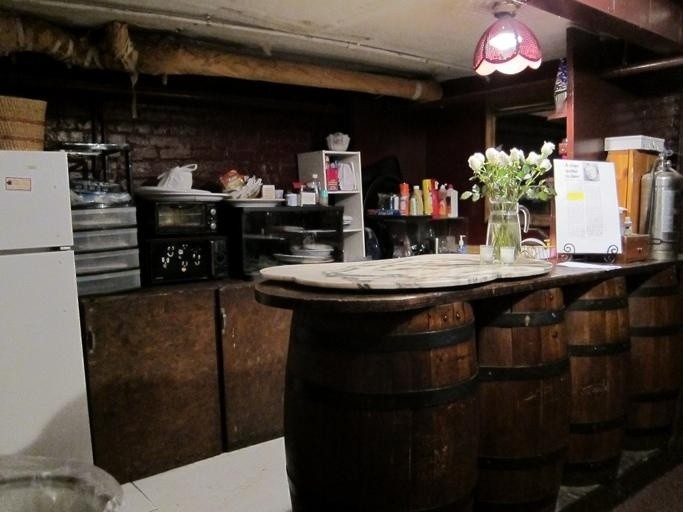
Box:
[[479, 244, 516, 268], [486, 201, 531, 260], [285, 194, 297, 207]]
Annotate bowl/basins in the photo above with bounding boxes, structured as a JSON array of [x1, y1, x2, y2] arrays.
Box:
[[326, 135, 349, 151], [291, 244, 333, 257]]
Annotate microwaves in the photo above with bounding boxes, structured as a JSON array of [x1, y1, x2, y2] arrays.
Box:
[[137, 200, 219, 237]]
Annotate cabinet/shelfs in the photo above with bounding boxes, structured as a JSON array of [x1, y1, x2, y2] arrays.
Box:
[[239, 147, 366, 282]]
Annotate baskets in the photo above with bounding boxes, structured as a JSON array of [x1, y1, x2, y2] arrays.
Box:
[[0, 95, 48, 151]]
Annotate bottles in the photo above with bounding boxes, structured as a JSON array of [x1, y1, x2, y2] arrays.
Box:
[[388, 179, 461, 219], [623, 215, 632, 235], [309, 173, 322, 203]]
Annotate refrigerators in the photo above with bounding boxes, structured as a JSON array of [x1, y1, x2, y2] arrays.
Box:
[[1, 148, 95, 467]]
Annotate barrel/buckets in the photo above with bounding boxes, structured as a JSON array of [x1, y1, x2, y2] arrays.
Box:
[[561, 275, 629, 487], [479, 288, 570, 510], [627, 264, 683, 451], [284, 300, 481, 511]]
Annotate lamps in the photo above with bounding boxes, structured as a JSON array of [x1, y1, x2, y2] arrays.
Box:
[[473, 1, 544, 77]]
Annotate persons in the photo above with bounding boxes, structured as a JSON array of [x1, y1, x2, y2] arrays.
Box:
[[583, 163, 600, 182]]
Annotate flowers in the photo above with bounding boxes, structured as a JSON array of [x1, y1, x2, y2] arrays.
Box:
[[464, 139, 557, 262]]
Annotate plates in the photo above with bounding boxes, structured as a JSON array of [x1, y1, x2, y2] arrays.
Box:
[[224, 198, 286, 209], [273, 254, 333, 264], [134, 185, 231, 202]]
